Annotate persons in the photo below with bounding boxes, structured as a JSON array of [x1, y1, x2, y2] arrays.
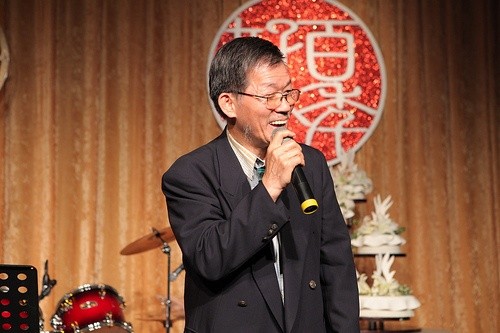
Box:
[[161, 37, 360, 333]]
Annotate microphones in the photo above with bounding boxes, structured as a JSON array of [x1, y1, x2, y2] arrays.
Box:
[[42, 275, 49, 296], [170, 265, 184, 281], [281, 136, 318, 215]]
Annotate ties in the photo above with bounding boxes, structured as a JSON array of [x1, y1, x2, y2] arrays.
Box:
[[255, 158, 265, 180]]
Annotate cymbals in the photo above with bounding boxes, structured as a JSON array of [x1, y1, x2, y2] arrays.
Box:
[[120, 227, 176, 256], [134, 309, 184, 321]]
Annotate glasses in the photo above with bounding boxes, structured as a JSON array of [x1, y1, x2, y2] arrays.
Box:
[[231, 89, 301, 110]]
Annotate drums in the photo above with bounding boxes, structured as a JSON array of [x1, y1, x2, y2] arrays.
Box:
[[51, 284, 134, 333]]
